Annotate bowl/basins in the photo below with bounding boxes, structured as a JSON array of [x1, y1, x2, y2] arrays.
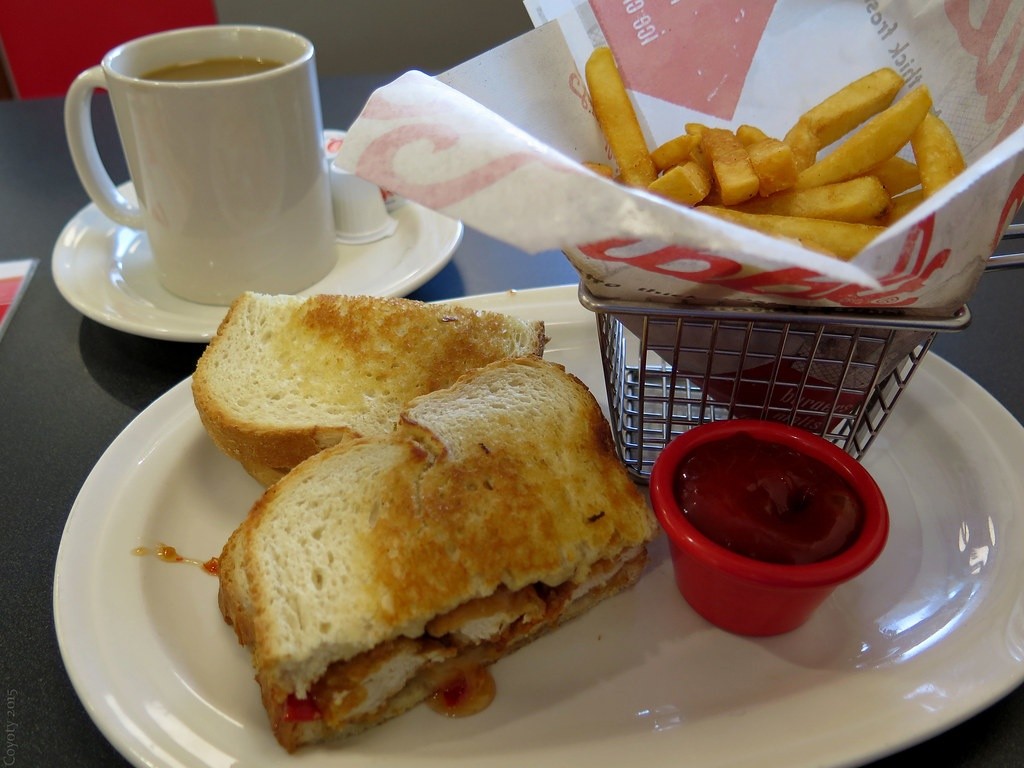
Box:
[[650, 418, 891, 634]]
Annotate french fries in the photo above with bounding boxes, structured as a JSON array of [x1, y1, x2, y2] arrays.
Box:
[[586, 49, 972, 264]]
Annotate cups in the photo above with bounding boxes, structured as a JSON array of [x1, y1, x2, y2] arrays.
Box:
[[64, 25, 337, 307]]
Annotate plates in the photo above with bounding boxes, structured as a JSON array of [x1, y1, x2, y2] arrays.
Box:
[[51, 180, 463, 344], [52, 283, 1024, 768]]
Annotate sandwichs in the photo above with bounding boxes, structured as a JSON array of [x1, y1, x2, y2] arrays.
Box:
[[193, 291, 656, 747]]
